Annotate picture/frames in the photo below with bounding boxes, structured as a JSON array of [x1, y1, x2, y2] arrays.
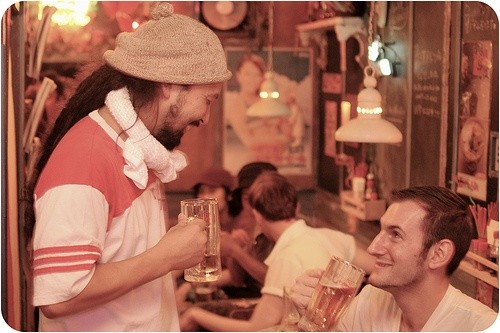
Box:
[[222, 38, 320, 182]]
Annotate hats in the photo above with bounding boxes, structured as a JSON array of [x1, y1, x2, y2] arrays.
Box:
[[191, 166, 233, 198], [103, 2, 232, 85], [200, 1, 248, 31]]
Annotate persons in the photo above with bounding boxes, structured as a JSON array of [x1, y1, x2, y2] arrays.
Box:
[[290, 185, 499, 332], [25, 2, 232, 332], [225, 54, 287, 146], [171, 161, 378, 332]]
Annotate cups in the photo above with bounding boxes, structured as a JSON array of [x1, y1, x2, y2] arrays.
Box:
[[297, 255, 366, 332], [181, 197, 222, 282], [352, 177, 365, 203]]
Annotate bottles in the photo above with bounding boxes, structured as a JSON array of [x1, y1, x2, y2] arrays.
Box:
[[365, 174, 376, 199]]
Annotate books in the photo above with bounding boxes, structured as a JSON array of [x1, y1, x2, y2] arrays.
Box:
[[21, 76, 57, 151], [26, 5, 56, 80]]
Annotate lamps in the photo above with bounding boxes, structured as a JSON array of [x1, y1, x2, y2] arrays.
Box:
[[245, 1, 291, 117], [335, 1, 403, 144]]
